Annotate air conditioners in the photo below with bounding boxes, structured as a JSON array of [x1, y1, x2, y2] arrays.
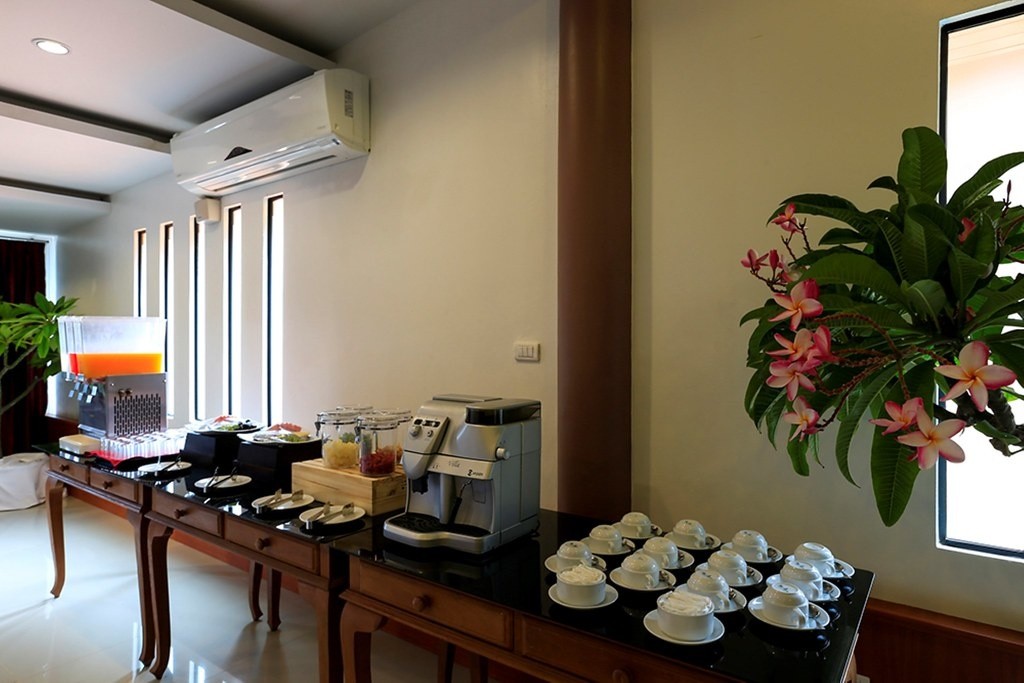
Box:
[[170, 68, 370, 202]]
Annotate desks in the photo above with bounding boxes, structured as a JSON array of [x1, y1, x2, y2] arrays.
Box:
[[31, 441, 875, 683]]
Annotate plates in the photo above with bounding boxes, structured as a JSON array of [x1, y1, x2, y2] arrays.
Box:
[[252, 493, 315, 511], [298, 505, 366, 525], [137, 461, 192, 472], [183, 416, 320, 445], [194, 474, 252, 488]]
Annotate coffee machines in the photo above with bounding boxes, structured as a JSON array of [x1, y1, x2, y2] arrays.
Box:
[[383, 393, 541, 569]]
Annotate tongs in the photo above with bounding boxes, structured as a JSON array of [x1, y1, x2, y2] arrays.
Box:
[[203, 466, 239, 493], [306, 500, 354, 530], [154, 454, 182, 477], [256, 488, 304, 514]]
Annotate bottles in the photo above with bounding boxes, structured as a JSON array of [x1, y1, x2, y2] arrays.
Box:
[[314, 404, 412, 478]]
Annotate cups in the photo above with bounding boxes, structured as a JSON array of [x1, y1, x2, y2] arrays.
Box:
[[545, 512, 855, 646]]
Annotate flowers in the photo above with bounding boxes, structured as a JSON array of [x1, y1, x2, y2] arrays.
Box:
[[737, 126, 1023, 528]]
